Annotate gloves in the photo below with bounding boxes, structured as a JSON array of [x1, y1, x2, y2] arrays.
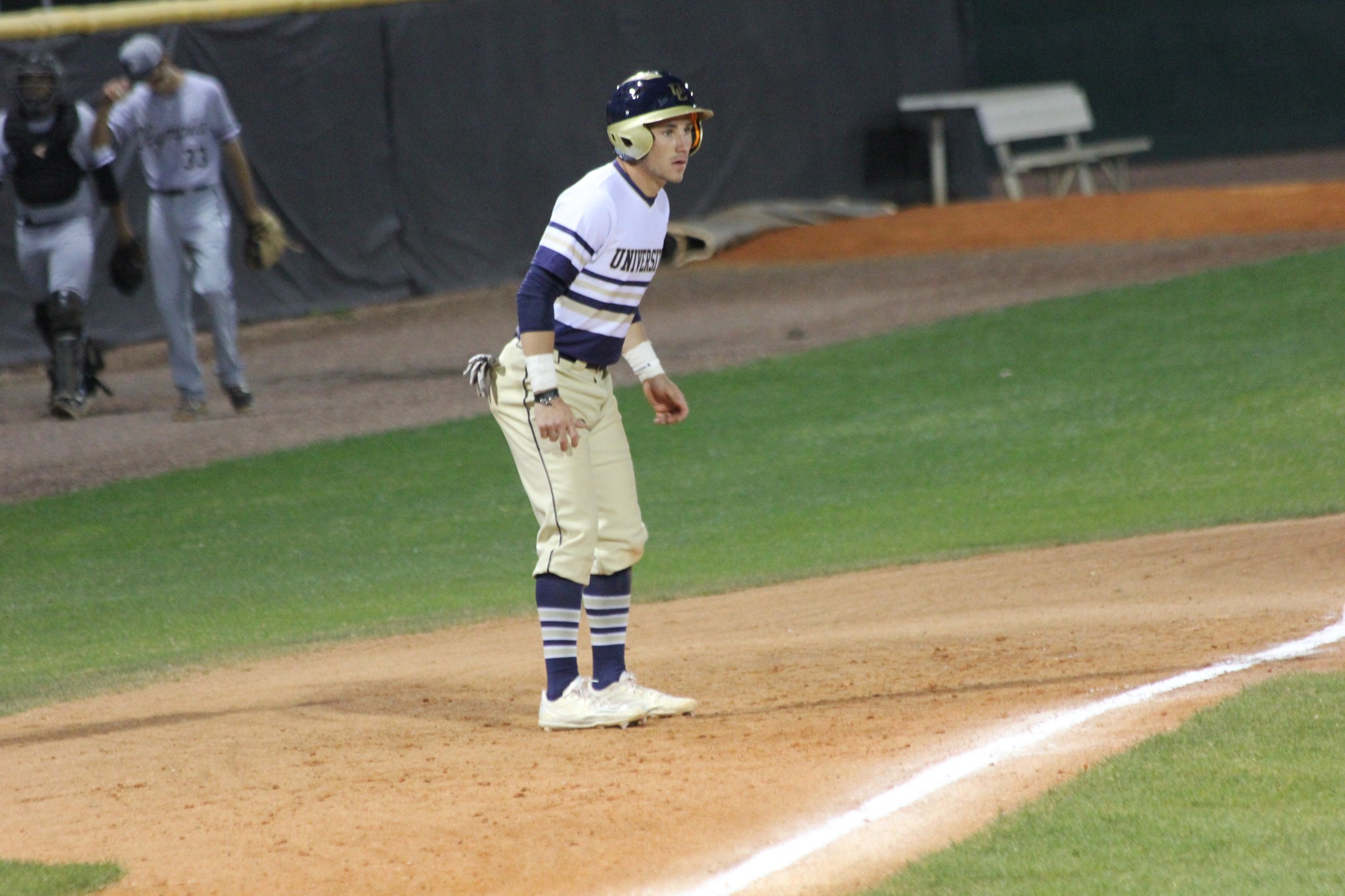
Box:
[[463, 353, 498, 398]]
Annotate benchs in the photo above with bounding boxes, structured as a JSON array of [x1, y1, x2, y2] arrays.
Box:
[[975, 82, 1154, 202]]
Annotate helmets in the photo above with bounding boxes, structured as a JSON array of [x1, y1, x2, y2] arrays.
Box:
[[15, 51, 63, 118], [605, 69, 713, 164]]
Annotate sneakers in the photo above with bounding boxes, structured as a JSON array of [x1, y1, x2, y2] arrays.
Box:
[[538, 675, 660, 732], [588, 671, 696, 719]]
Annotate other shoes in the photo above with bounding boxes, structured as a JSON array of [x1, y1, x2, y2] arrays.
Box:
[[227, 386, 254, 411], [53, 398, 80, 418], [174, 398, 207, 422]]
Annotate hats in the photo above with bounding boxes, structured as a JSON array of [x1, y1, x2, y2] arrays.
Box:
[[120, 36, 164, 88]]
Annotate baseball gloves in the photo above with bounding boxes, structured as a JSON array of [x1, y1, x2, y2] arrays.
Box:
[[107, 241, 146, 296], [242, 207, 287, 272]]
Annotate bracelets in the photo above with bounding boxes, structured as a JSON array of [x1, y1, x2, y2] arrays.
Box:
[[621, 340, 665, 383], [524, 353, 558, 393], [534, 388, 559, 405]]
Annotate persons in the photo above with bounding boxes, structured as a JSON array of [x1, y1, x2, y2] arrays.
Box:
[[463, 69, 714, 729], [0, 55, 138, 419], [85, 34, 269, 423]]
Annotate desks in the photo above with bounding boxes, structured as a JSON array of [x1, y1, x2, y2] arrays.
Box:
[[898, 88, 1005, 204]]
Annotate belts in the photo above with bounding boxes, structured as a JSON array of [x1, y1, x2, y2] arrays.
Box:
[[514, 333, 607, 370], [150, 184, 209, 198], [21, 219, 62, 227]]
